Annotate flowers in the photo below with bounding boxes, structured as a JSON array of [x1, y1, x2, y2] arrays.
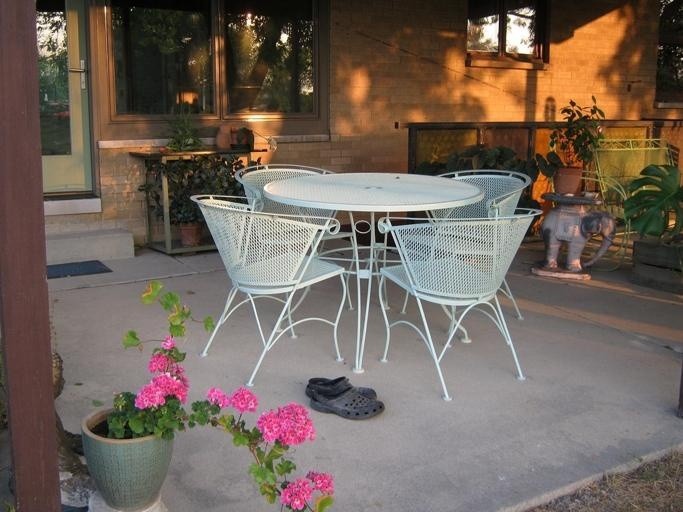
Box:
[[105, 279, 335, 512]]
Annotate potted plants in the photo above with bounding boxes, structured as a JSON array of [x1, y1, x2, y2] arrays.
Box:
[[137, 153, 265, 247], [228, 127, 255, 151], [535, 93, 605, 196], [622, 163, 683, 294]]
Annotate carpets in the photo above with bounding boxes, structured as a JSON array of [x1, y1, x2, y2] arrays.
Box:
[[46, 259, 114, 280]]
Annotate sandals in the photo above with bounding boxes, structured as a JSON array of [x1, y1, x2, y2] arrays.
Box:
[[304, 375, 378, 401], [309, 387, 386, 420]]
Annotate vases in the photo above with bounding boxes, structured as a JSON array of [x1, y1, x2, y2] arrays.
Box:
[[80, 405, 175, 512]]
